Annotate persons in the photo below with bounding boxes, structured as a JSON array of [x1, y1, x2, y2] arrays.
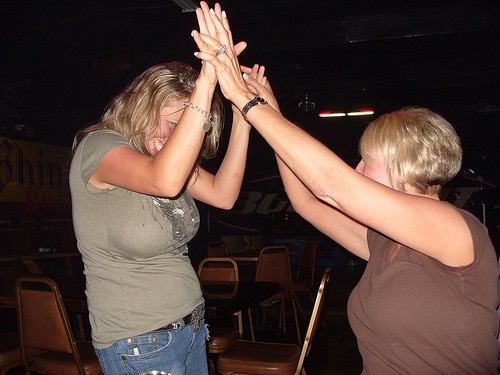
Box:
[[65, 0, 268, 375], [194, 8, 500, 375]]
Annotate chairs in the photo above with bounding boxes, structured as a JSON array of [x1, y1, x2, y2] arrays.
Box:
[[0, 239, 335, 375]]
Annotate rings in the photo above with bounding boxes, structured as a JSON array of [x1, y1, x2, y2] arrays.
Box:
[[215, 44, 228, 55]]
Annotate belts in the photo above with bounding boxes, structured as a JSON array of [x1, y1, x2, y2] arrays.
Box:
[[159, 302, 206, 330]]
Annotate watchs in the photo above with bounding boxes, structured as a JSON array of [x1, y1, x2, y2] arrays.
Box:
[[241, 95, 267, 121]]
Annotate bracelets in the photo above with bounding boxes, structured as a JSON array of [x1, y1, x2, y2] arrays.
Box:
[[182, 101, 212, 132]]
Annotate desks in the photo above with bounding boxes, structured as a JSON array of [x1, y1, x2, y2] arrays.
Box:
[[0, 273, 88, 341], [199, 278, 288, 340]]
